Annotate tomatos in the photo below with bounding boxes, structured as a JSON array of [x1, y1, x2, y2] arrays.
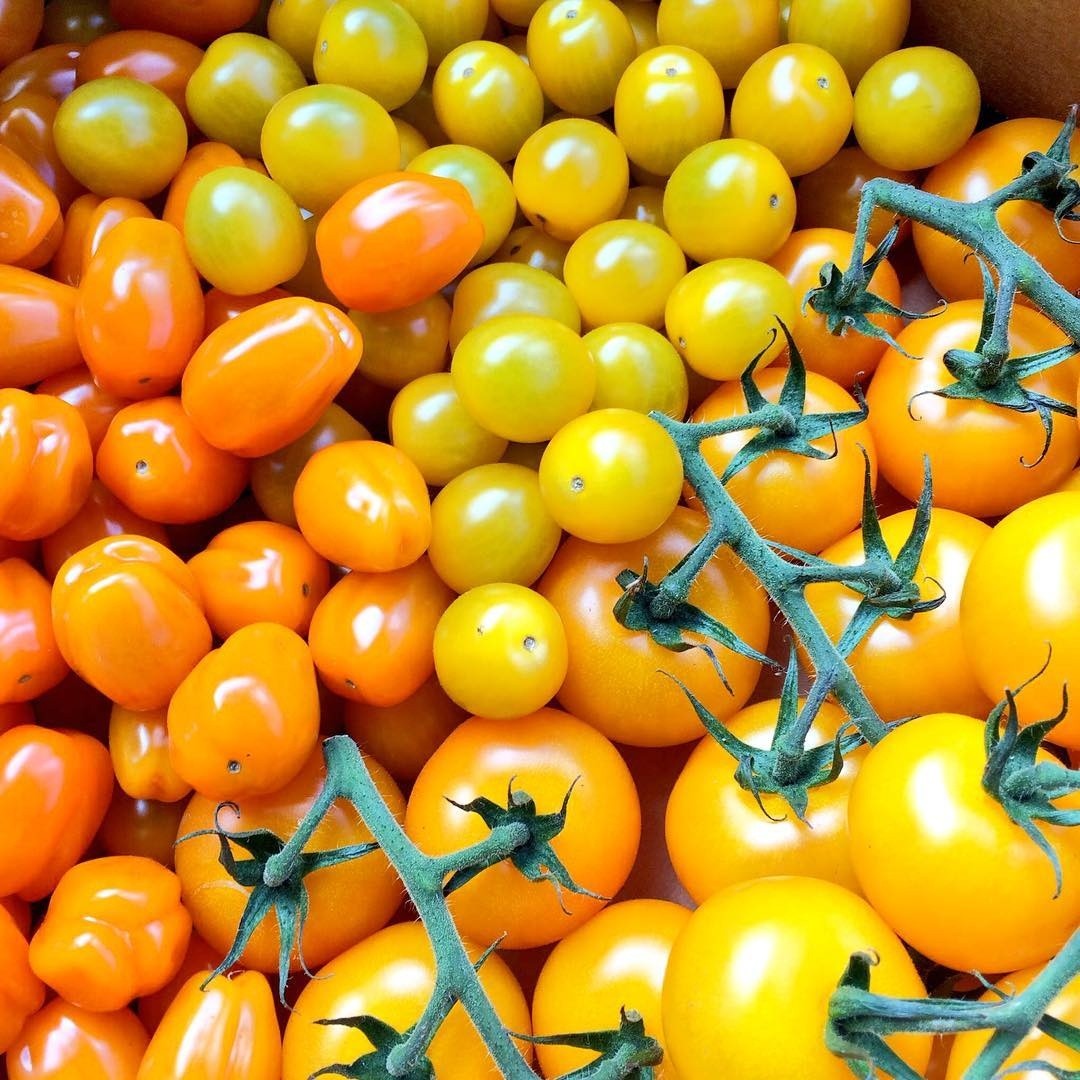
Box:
[[0, 0, 1080, 1080]]
